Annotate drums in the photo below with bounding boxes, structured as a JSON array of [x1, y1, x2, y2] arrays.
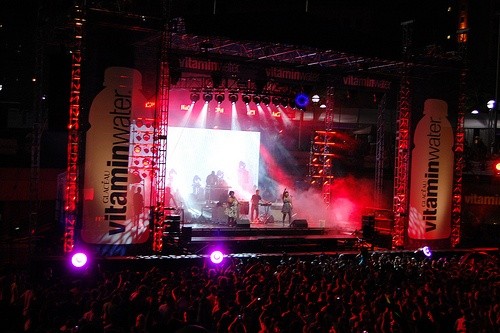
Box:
[[238, 200, 249, 216]]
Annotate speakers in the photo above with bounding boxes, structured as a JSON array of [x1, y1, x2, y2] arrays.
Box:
[[362, 216, 374, 233], [290, 220, 308, 228], [182, 227, 192, 240], [235, 219, 250, 227]]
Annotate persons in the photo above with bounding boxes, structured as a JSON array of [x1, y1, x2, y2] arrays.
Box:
[[0, 245, 500, 333], [251, 190, 263, 223], [281, 187, 293, 226], [206, 171, 219, 205], [127, 186, 145, 228], [161, 187, 178, 215], [226, 191, 242, 227]]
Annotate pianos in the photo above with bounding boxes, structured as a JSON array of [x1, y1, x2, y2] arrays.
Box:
[[258, 199, 274, 224], [269, 203, 293, 222]]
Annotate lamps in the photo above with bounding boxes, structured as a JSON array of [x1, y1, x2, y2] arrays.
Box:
[[289, 95, 306, 112], [189, 90, 200, 103], [271, 94, 281, 108], [252, 93, 262, 106], [214, 91, 224, 103], [133, 120, 159, 179], [279, 95, 289, 109], [310, 132, 332, 186], [202, 91, 213, 104], [242, 93, 252, 105], [228, 92, 238, 105], [262, 93, 271, 107], [417, 245, 432, 256]]
